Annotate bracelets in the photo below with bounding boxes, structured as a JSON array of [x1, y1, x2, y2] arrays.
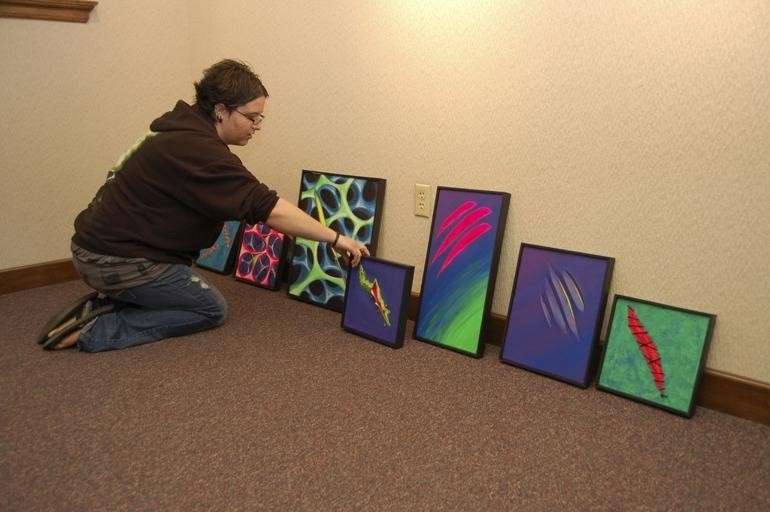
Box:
[[332, 232, 340, 247]]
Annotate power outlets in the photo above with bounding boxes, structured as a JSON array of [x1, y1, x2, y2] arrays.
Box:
[[412, 183, 433, 220]]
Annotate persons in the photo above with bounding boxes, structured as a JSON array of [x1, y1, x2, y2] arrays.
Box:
[[37, 59, 370, 352]]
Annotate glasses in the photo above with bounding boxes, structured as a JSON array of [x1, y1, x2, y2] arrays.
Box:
[[231, 106, 265, 125]]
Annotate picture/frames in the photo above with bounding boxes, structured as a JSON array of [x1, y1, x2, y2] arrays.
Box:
[[594, 294, 718, 419], [231, 218, 286, 291], [193, 218, 242, 275], [411, 185, 512, 360], [498, 242, 616, 391], [286, 169, 387, 314], [341, 256, 416, 350]]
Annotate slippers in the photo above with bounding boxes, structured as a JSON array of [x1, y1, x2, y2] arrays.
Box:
[[37, 291, 115, 349]]
